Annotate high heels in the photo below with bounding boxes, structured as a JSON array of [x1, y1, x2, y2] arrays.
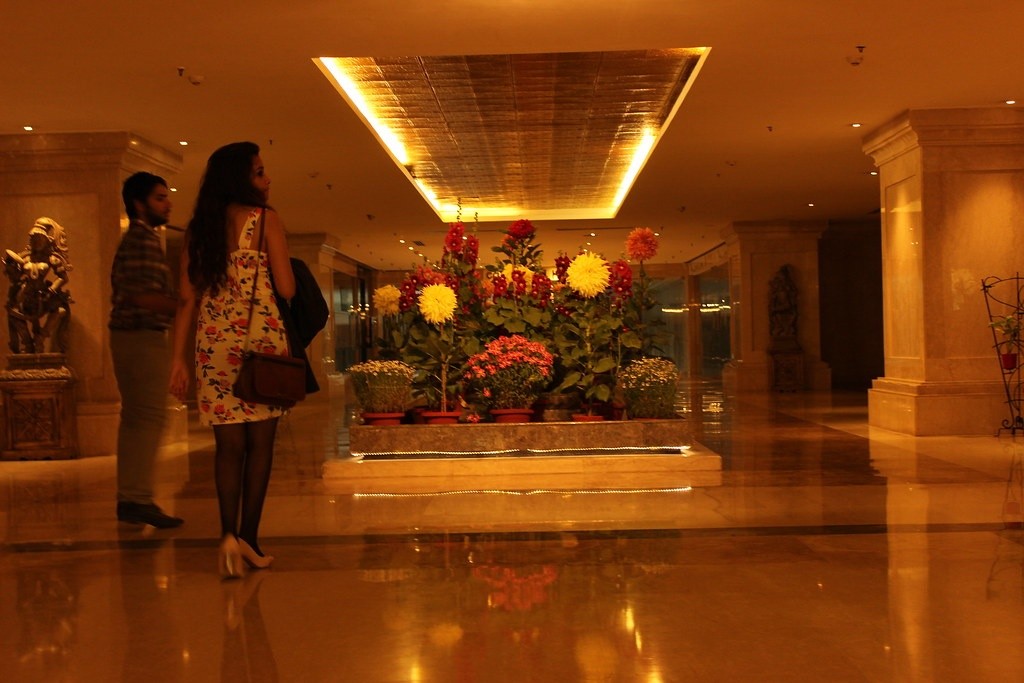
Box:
[[238, 540, 274, 567], [217, 534, 243, 580]]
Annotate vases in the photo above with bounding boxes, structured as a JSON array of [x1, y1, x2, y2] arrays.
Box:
[[411, 408, 426, 424], [572, 409, 605, 422], [610, 396, 625, 421], [544, 391, 569, 411], [421, 411, 462, 424], [361, 411, 406, 425], [489, 407, 535, 423]]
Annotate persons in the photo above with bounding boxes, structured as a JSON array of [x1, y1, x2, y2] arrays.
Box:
[[168, 140, 306, 581], [0, 216, 75, 355], [108, 171, 187, 531], [767, 265, 799, 336]]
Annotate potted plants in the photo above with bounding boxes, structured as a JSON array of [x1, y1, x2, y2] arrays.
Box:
[[987, 315, 1020, 370]]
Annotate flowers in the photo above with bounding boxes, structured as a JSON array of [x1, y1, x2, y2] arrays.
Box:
[[345, 192, 687, 422]]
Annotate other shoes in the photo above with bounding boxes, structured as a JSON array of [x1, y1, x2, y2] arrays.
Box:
[[117, 500, 185, 529]]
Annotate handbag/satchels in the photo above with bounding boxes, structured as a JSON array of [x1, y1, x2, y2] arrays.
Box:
[[234, 353, 308, 409]]
